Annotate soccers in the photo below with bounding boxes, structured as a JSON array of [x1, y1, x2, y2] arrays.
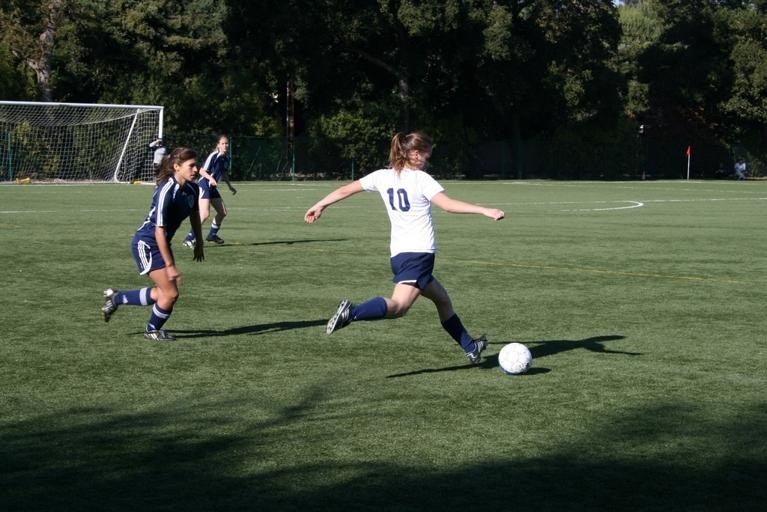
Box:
[[499, 342, 533, 374]]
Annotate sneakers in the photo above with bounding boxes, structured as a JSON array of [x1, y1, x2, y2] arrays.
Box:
[[206, 235, 224, 244], [144, 326, 176, 341], [183, 239, 196, 249], [326, 300, 355, 335], [466, 334, 488, 364], [101, 287, 119, 322]]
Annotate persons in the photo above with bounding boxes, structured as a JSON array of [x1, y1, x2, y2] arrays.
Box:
[[147, 135, 168, 191], [732, 158, 747, 180], [101, 146, 205, 342], [712, 162, 726, 178], [304, 129, 503, 365], [181, 135, 237, 253]]
